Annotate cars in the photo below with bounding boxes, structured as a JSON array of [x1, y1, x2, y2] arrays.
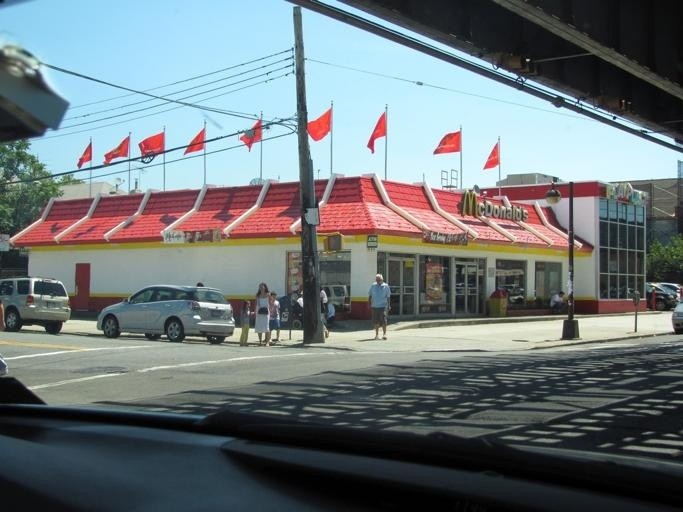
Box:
[[670, 300, 682, 333], [96, 284, 234, 344], [645, 282, 682, 311], [455, 282, 474, 289]]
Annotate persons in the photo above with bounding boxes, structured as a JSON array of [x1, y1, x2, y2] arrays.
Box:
[[240, 300, 255, 346], [197, 282, 203, 286], [268, 291, 281, 342], [320, 287, 330, 338], [550, 291, 568, 314], [368, 273, 391, 340], [253, 282, 270, 346]]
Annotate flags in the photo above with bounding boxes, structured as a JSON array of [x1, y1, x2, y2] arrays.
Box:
[[104, 136, 129, 164], [183, 129, 204, 155], [483, 142, 499, 169], [306, 108, 332, 142], [77, 143, 92, 169], [240, 120, 261, 152], [138, 132, 165, 158], [434, 131, 461, 154], [368, 112, 387, 154]]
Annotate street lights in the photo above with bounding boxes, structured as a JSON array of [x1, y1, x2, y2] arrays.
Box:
[[544, 179, 581, 339]]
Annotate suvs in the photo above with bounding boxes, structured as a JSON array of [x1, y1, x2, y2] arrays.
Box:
[[320, 282, 350, 312], [0, 275, 72, 335]]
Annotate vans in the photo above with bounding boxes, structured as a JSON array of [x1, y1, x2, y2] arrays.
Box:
[[503, 284, 520, 295]]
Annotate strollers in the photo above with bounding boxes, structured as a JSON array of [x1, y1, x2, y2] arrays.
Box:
[[292, 297, 303, 329]]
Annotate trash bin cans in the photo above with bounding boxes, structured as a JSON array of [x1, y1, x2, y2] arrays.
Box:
[[488, 290, 508, 317]]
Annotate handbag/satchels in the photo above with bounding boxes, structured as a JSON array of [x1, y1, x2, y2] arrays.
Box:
[[257, 307, 269, 315]]
